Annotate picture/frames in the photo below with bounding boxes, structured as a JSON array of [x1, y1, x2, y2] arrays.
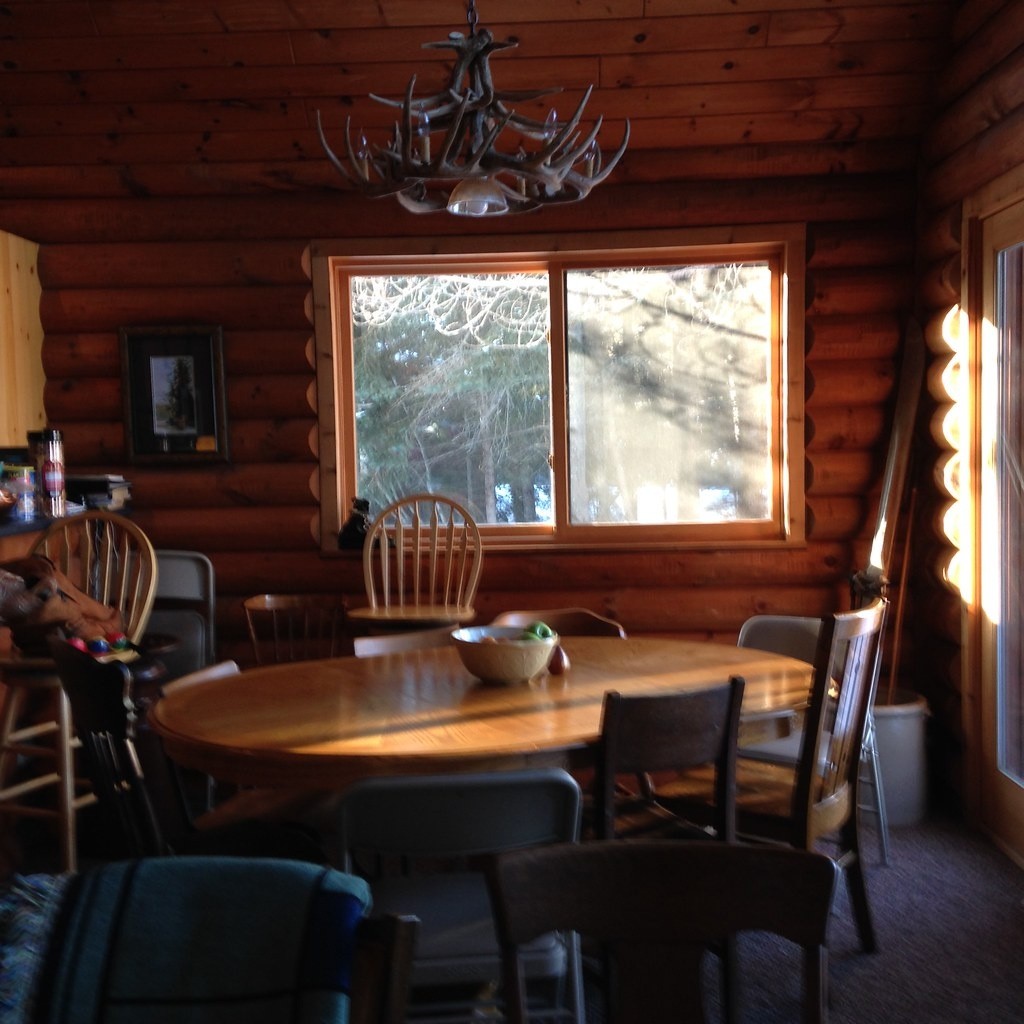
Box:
[[119, 323, 230, 468]]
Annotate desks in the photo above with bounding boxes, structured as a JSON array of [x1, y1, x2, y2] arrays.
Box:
[[0, 506, 137, 788], [147, 637, 817, 789]]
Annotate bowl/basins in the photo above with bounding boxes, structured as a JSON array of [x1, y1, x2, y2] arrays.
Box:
[[450, 625, 559, 684]]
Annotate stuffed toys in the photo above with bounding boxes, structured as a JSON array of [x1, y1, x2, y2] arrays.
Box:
[[0, 553, 74, 651]]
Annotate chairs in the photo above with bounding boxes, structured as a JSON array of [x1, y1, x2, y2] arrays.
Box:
[[0, 854, 424, 1024], [348, 494, 481, 635], [737, 615, 892, 867], [594, 675, 746, 1024], [489, 608, 628, 638], [0, 511, 159, 875], [195, 593, 347, 828], [329, 764, 587, 1024], [485, 839, 840, 1024], [93, 549, 215, 682]]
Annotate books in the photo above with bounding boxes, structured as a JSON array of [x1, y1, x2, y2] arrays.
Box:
[[63, 474, 133, 511]]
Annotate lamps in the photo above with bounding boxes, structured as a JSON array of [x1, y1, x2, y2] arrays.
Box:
[[315, 1, 630, 219]]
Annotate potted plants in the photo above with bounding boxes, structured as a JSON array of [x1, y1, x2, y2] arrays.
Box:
[[845, 569, 929, 829]]
